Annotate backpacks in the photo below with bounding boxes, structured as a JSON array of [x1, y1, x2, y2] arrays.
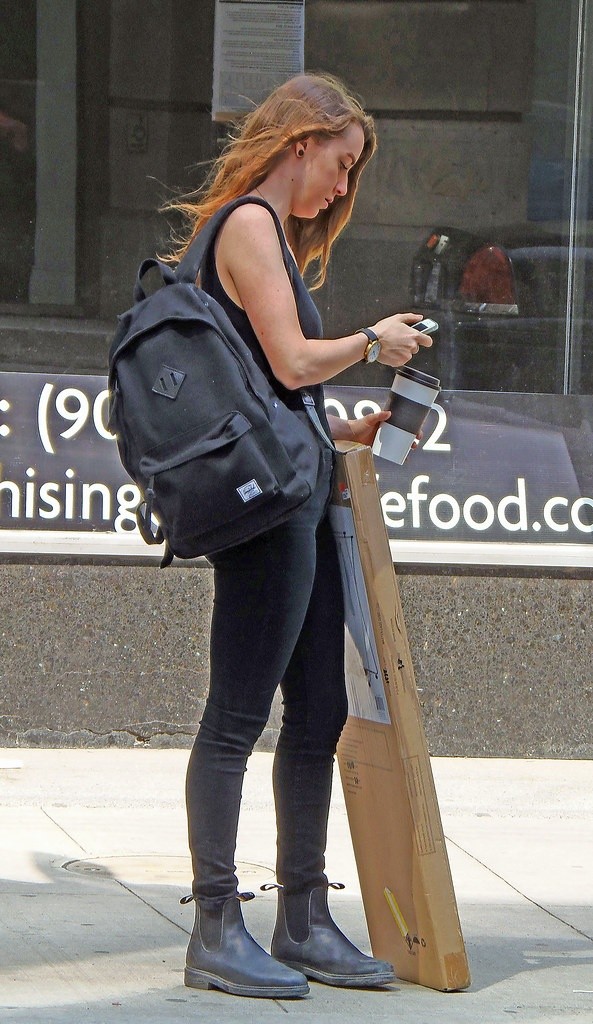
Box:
[[106, 195, 349, 569]]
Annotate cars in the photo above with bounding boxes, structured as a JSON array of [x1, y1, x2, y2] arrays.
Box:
[[388, 222, 591, 396]]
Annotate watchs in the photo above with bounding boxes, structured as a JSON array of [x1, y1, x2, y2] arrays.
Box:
[[354, 327, 380, 364]]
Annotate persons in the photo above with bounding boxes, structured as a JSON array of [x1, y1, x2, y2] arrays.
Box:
[[185, 76, 433, 998]]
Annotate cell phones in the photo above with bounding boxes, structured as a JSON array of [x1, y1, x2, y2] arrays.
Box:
[[411, 317, 439, 334]]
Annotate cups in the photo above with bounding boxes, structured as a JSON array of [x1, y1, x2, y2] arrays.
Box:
[[371, 364, 442, 466]]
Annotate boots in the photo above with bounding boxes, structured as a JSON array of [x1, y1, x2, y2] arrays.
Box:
[[179, 890, 311, 998], [261, 880, 396, 984]]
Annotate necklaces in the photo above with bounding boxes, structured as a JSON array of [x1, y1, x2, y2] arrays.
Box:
[[256, 188, 263, 200]]
